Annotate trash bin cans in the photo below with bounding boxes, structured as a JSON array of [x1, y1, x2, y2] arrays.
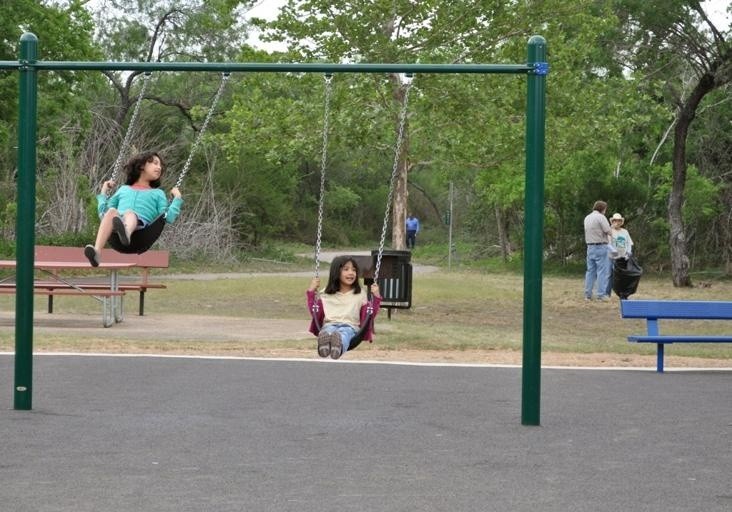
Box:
[[369, 250, 413, 318]]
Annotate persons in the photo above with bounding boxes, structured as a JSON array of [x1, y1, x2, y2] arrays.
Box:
[[606, 213, 634, 298], [84, 150, 184, 267], [584, 201, 612, 302], [307, 256, 383, 359], [406, 213, 419, 249]]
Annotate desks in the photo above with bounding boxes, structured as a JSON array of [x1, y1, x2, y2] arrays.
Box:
[[0, 259, 136, 330]]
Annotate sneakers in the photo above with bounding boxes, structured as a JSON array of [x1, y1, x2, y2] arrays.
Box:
[[112, 216, 131, 246], [84, 245, 99, 267], [584, 296, 593, 302], [595, 297, 610, 303], [330, 331, 341, 359], [318, 330, 330, 357]]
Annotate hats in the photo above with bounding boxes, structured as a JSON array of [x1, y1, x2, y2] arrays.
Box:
[[609, 213, 624, 226]]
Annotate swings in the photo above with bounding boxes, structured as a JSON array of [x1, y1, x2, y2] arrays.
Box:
[[313, 74, 411, 351], [102, 73, 231, 254]]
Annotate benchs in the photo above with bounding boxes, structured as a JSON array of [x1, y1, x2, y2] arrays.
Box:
[[29, 245, 169, 316], [618, 298, 732, 374], [0, 284, 142, 322], [0, 287, 122, 329]]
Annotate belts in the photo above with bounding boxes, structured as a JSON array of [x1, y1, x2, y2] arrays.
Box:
[[587, 242, 607, 245]]
